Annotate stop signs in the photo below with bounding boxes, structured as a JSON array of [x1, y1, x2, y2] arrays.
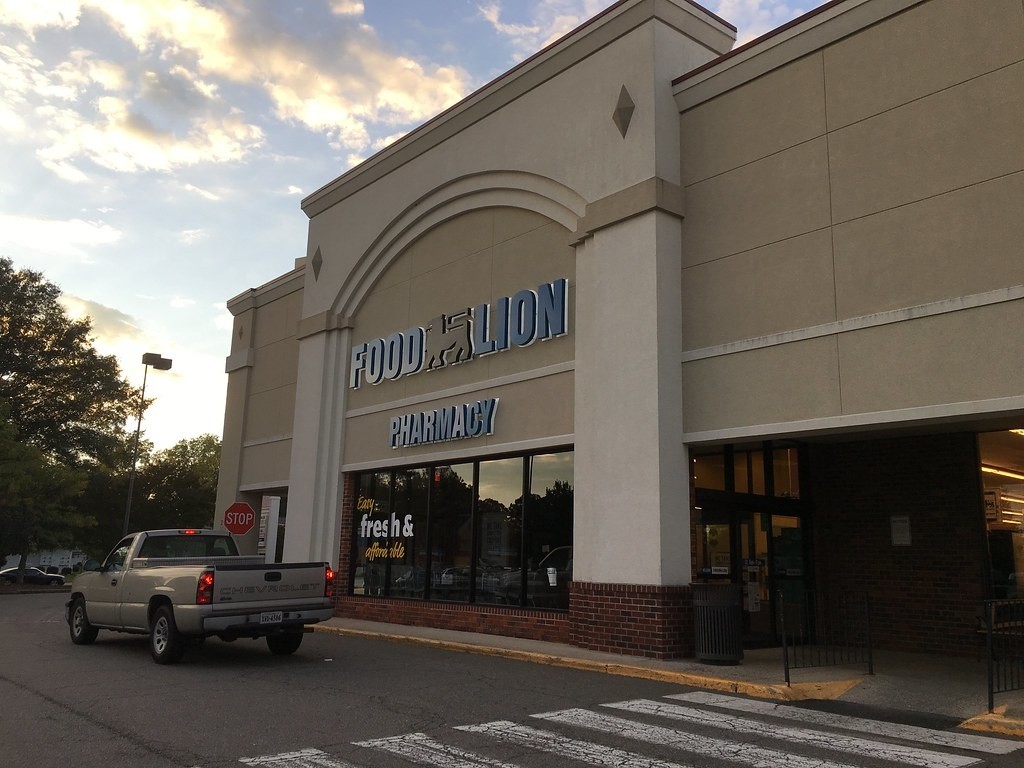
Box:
[[224, 502, 257, 535]]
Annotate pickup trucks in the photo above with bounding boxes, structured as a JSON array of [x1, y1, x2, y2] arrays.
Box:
[[65, 528, 336, 665]]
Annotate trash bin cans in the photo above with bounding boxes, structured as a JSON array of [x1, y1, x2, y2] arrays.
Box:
[[689, 581, 747, 665]]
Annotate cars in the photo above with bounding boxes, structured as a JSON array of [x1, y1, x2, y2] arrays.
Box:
[[0, 567, 66, 587]]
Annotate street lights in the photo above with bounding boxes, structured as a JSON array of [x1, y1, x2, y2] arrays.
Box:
[[120, 353, 172, 538]]
